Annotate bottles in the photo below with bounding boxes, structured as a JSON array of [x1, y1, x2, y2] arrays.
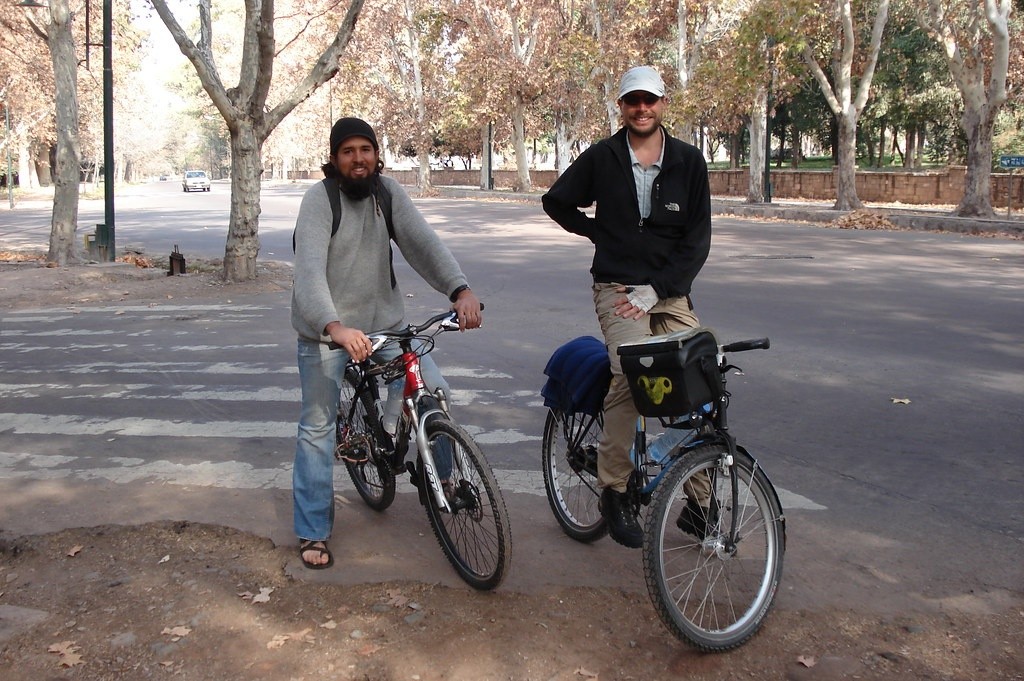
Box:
[[647, 403, 711, 467], [395, 403, 411, 444], [383, 376, 405, 437]]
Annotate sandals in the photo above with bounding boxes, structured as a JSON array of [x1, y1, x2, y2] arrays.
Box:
[[440, 479, 455, 498], [300, 537, 333, 569]]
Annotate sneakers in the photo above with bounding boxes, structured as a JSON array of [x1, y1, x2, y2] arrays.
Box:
[[598, 485, 645, 549], [677, 496, 722, 541]]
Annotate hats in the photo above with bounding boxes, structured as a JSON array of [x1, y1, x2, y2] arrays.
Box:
[[617, 66, 665, 98], [330, 117, 378, 155]]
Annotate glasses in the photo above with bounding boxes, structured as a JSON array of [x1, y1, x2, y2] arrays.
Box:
[[621, 93, 659, 105]]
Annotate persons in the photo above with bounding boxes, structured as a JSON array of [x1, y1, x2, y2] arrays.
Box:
[[542, 66, 737, 556], [290, 117, 481, 569]]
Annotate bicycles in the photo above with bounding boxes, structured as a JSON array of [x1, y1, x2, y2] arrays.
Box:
[[541, 328, 787, 655], [327, 302, 514, 592]]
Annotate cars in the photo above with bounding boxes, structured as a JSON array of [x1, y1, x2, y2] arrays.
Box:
[[183, 170, 210, 192]]
